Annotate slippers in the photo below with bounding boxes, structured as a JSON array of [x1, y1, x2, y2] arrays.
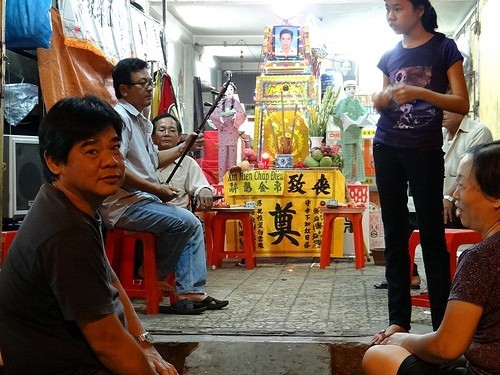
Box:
[[375, 280, 421, 288], [160, 298, 206, 314], [194, 296, 228, 309]]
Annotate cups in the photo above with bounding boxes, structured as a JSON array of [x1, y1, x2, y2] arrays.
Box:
[[246, 202, 255, 208], [328, 200, 338, 206]]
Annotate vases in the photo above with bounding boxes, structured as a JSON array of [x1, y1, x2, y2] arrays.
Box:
[[309, 137, 324, 149]]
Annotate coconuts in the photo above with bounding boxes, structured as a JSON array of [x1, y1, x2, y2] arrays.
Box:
[[303, 150, 332, 167]]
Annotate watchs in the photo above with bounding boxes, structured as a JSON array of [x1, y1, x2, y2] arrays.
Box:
[[444, 194, 455, 203], [136, 332, 154, 343]]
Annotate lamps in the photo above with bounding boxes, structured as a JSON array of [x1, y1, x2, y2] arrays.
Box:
[[199, 54, 217, 69]]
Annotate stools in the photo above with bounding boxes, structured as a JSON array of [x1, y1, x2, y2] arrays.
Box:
[[320, 213, 366, 270], [194, 212, 256, 270], [408, 228, 483, 307], [104, 231, 176, 313]]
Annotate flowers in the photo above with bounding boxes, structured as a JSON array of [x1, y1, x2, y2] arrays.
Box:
[[297, 76, 341, 137]]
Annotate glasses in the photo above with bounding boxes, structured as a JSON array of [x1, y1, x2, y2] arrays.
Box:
[[127, 81, 157, 88]]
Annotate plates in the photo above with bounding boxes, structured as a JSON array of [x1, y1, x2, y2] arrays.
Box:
[[310, 167, 339, 169]]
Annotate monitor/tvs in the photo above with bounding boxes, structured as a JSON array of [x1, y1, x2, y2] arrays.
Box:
[[4, 134, 48, 220]]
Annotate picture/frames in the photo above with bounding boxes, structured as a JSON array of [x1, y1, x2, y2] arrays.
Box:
[[273, 26, 299, 61], [333, 53, 359, 86]]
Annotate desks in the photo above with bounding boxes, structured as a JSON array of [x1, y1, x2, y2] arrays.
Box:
[[223, 170, 345, 264]]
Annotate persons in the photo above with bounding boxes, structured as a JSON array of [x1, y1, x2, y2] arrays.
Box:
[[277, 29, 297, 55], [97, 57, 229, 313], [361, 139, 500, 375], [374, 88, 494, 295], [371, 0, 470, 344], [151, 112, 215, 209], [0, 93, 179, 375], [340, 60, 355, 81]]
